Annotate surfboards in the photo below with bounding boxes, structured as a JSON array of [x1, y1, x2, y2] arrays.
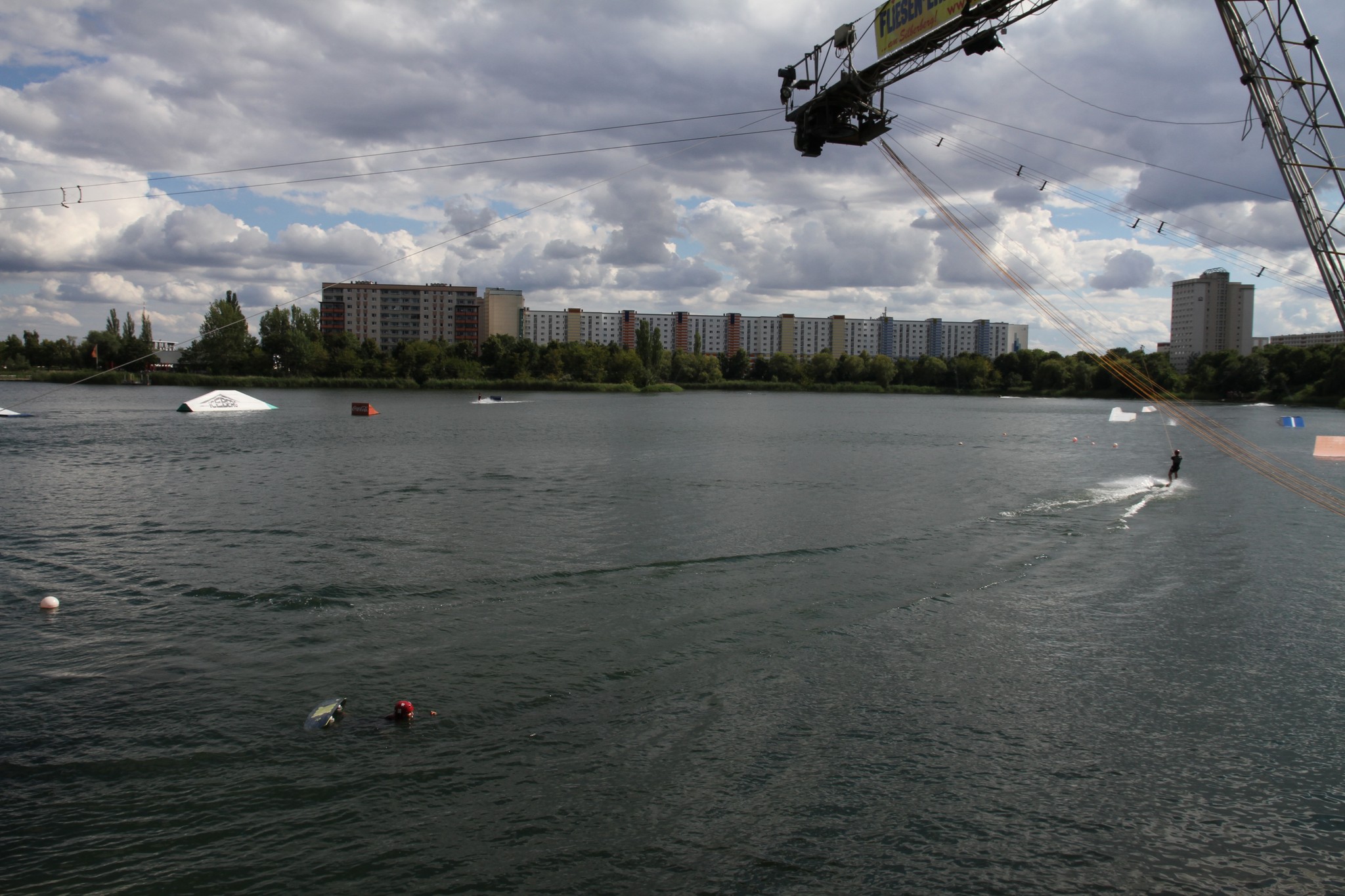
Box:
[[302, 696, 348, 733]]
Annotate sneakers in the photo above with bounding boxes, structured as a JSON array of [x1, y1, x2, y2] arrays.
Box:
[[326, 716, 335, 727], [336, 704, 343, 712]]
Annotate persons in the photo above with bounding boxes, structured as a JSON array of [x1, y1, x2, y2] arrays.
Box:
[[1168, 449, 1183, 483], [328, 701, 436, 725], [478, 393, 481, 402]]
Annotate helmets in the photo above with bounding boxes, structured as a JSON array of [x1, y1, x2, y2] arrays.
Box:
[[1175, 449, 1180, 455], [394, 701, 414, 713]]
